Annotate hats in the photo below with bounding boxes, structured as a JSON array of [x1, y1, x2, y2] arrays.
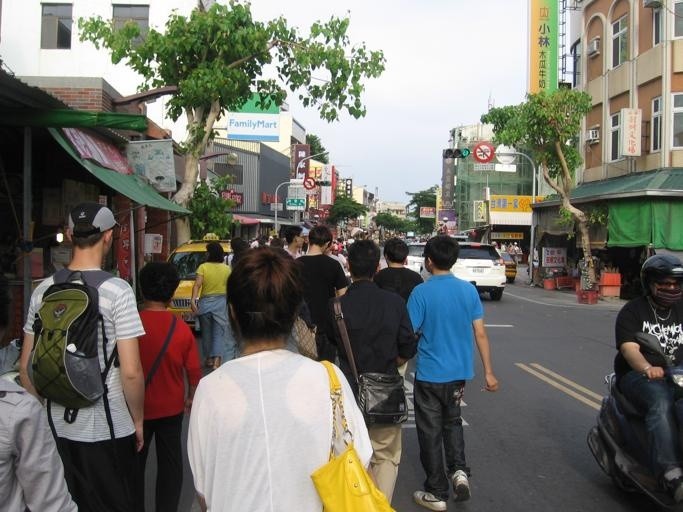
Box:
[[69, 203, 120, 238]]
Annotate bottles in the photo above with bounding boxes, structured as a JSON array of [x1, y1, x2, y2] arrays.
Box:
[[66, 343, 104, 400]]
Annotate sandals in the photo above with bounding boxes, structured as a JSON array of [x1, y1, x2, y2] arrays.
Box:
[[205, 360, 213, 367], [212, 365, 218, 370]]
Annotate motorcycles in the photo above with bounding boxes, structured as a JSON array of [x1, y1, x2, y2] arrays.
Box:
[[586, 332, 682, 512]]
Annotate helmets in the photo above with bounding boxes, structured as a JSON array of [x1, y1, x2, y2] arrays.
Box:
[[640, 254, 683, 308]]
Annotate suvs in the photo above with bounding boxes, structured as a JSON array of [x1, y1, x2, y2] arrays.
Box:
[[403, 242, 507, 301]]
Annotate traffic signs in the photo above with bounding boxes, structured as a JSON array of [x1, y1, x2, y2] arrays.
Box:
[[286, 199, 305, 206]]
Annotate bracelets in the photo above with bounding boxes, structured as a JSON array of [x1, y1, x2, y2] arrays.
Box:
[[641, 366, 651, 377]]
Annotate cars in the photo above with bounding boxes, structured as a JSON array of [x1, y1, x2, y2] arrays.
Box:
[[166, 233, 235, 328]]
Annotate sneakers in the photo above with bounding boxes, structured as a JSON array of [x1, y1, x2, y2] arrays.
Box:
[[413, 490, 447, 512], [451, 469, 471, 503], [662, 467, 683, 504]]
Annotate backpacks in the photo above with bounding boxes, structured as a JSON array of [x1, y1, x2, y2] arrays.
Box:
[[26, 266, 118, 410]]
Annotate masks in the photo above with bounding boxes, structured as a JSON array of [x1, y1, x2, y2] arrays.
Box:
[[651, 283, 683, 305]]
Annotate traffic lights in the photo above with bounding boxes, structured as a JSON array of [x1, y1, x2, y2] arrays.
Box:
[[443, 148, 471, 158], [316, 181, 331, 186]]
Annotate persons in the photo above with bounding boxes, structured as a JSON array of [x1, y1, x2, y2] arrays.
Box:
[[282, 227, 305, 260], [406, 234, 499, 512], [297, 237, 305, 256], [372, 237, 424, 303], [305, 236, 310, 256], [187, 243, 376, 512], [19, 204, 146, 511], [315, 240, 418, 504], [131, 262, 202, 511], [327, 237, 350, 276], [246, 233, 281, 248], [189, 244, 233, 371], [613, 254, 683, 503], [351, 230, 405, 240], [491, 241, 520, 252], [294, 227, 349, 366], [0, 274, 78, 512], [222, 237, 244, 265]]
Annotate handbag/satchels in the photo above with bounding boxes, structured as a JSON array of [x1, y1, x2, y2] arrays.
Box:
[[288, 316, 318, 360], [310, 446, 396, 512], [357, 372, 408, 425]]
